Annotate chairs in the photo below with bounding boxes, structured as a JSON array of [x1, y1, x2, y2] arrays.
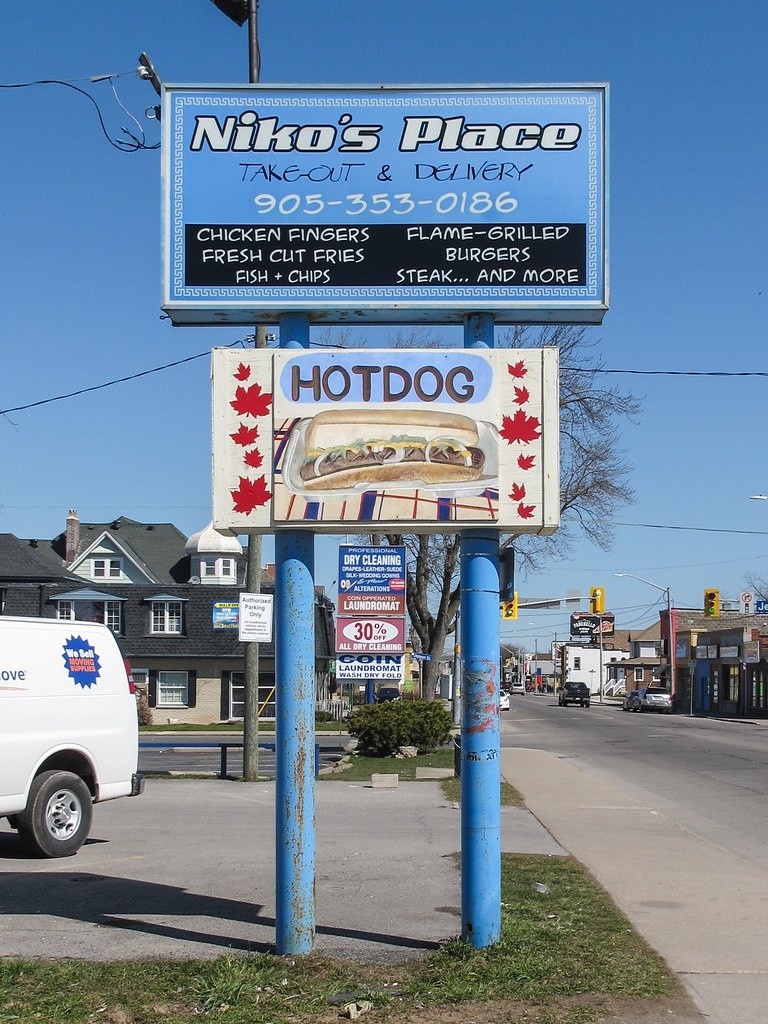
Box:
[[566, 684, 571, 688]]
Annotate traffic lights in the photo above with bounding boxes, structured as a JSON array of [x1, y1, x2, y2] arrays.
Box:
[[703, 588, 720, 618], [561, 646, 568, 674], [502, 591, 518, 619]]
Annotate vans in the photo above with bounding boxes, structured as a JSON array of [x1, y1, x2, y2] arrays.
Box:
[[0, 613, 145, 857]]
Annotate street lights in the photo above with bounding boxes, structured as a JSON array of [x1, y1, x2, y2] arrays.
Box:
[[612, 573, 678, 714]]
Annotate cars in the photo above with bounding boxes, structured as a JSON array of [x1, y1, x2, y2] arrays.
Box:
[[558, 682, 590, 708], [509, 682, 525, 696], [374, 687, 401, 705], [499, 689, 510, 711], [622, 685, 673, 714]]
